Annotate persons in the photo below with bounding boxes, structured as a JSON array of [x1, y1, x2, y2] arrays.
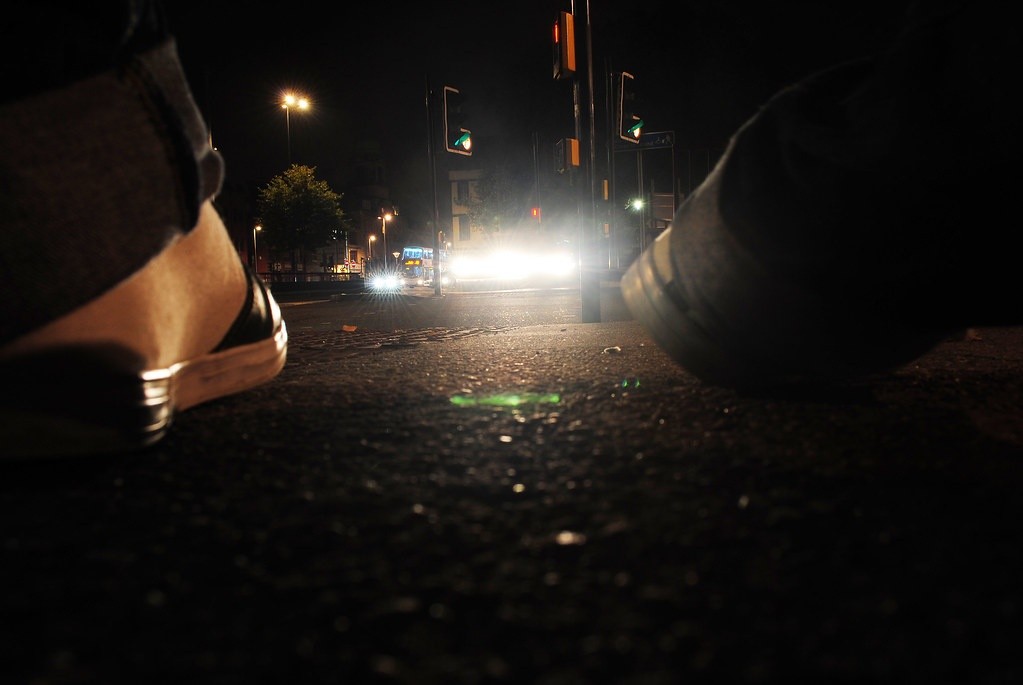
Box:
[[0, 0, 1022, 454]]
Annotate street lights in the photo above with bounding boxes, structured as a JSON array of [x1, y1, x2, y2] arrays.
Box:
[[280, 90, 312, 167], [253, 226, 263, 277], [381, 214, 391, 274], [393, 251, 400, 267], [368, 236, 376, 264]]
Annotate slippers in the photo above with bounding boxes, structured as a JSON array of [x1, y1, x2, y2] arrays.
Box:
[[615, 221, 943, 387], [0, 263, 288, 454]]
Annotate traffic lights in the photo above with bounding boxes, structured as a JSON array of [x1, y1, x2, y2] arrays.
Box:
[[620, 71, 642, 144], [442, 87, 474, 156]]
